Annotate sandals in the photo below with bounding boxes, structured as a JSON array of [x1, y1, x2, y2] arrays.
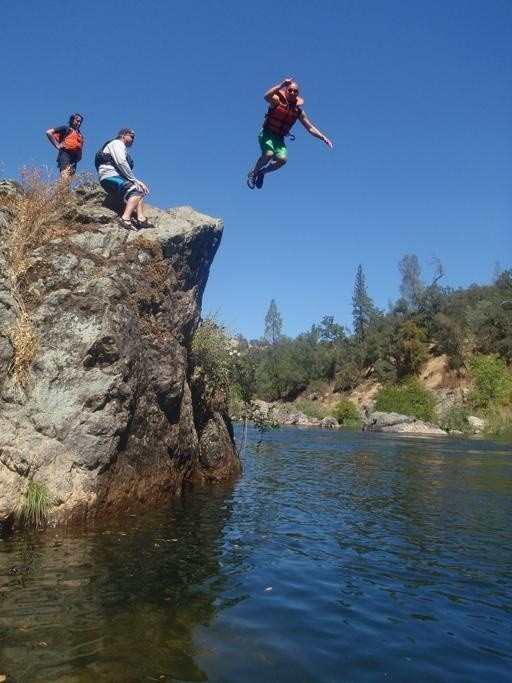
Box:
[[117, 215, 154, 230]]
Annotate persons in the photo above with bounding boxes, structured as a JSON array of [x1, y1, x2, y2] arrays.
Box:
[[95, 126, 156, 230], [246, 74, 334, 189], [45, 113, 84, 189]]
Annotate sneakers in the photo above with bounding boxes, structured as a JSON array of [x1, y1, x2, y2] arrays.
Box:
[[247, 169, 265, 188]]
